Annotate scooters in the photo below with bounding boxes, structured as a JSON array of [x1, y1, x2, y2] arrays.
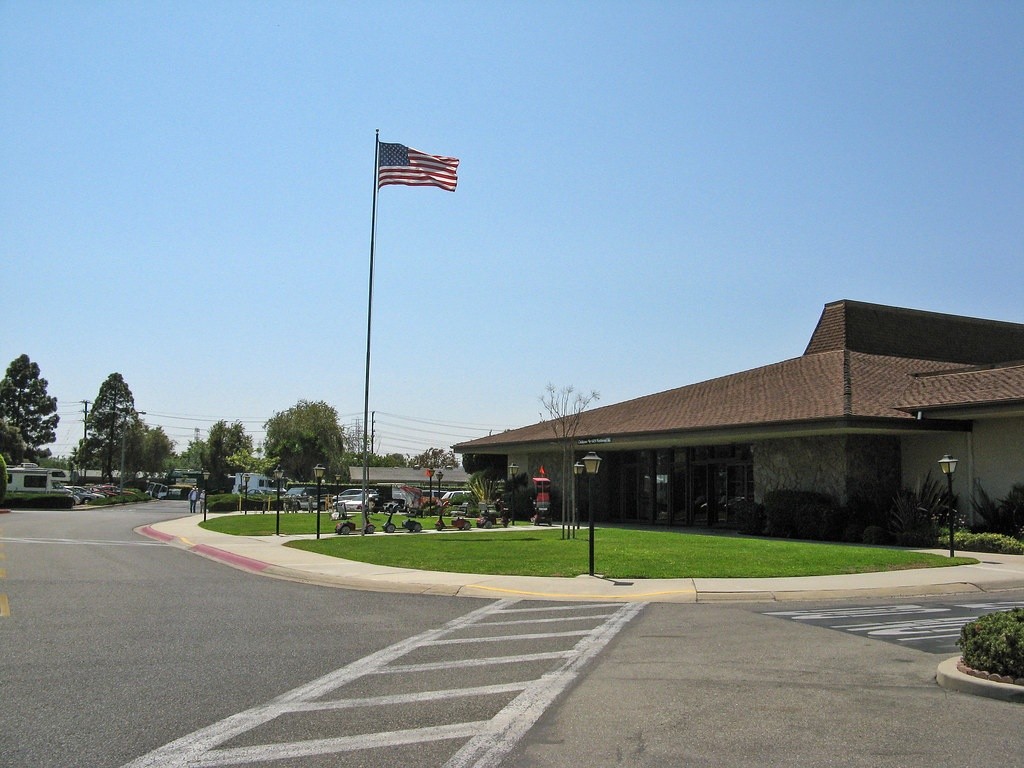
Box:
[[335, 507, 376, 534], [434, 506, 472, 531], [381, 506, 423, 533], [531, 501, 555, 526], [476, 506, 511, 528]]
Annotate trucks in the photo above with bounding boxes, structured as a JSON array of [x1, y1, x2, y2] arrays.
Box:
[[7, 462, 71, 492], [235, 473, 287, 493]]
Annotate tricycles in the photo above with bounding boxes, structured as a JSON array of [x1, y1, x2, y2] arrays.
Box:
[[145, 482, 168, 500]]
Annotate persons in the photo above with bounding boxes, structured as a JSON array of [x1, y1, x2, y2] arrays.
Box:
[[188, 487, 199, 513], [199, 490, 205, 513]]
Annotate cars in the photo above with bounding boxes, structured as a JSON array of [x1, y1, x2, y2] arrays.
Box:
[[64, 485, 136, 505]]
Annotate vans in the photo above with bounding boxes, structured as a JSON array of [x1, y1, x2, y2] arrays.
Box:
[[282, 486, 383, 512], [421, 489, 472, 498]]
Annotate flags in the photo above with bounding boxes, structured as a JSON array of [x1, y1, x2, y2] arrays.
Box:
[[378, 142, 459, 192]]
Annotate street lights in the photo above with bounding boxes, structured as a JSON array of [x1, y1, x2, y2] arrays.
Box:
[[937, 453, 959, 557], [243, 473, 251, 515], [572, 461, 585, 529], [313, 464, 325, 539], [273, 464, 284, 535], [120, 411, 146, 496], [435, 471, 444, 498], [427, 467, 435, 517], [202, 469, 211, 522], [582, 452, 602, 575], [334, 472, 341, 512], [508, 462, 520, 525]]
